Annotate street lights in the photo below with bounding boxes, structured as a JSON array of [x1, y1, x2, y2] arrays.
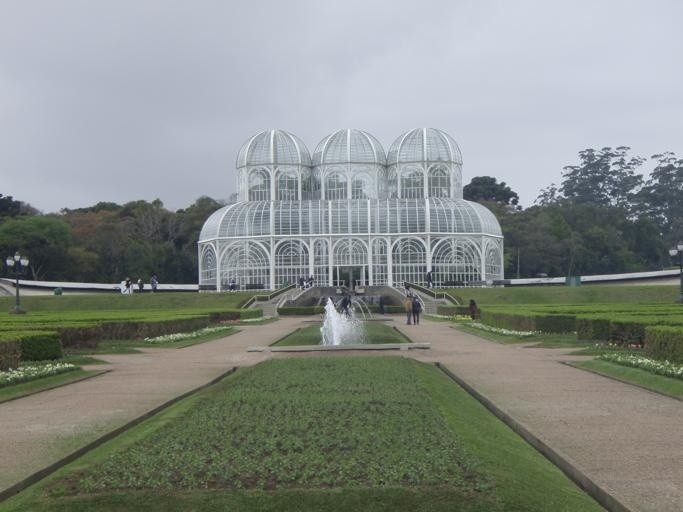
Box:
[[669, 241, 682, 303], [4, 252, 28, 313]]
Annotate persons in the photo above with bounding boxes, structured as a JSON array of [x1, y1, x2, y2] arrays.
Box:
[[469, 298, 479, 323], [425, 270, 434, 289], [411, 297, 421, 325], [136, 278, 144, 293], [308, 275, 314, 286], [404, 282, 410, 297], [228, 279, 236, 293], [299, 276, 306, 290], [150, 272, 158, 294], [403, 295, 413, 325], [123, 276, 130, 295], [378, 295, 384, 314]]
